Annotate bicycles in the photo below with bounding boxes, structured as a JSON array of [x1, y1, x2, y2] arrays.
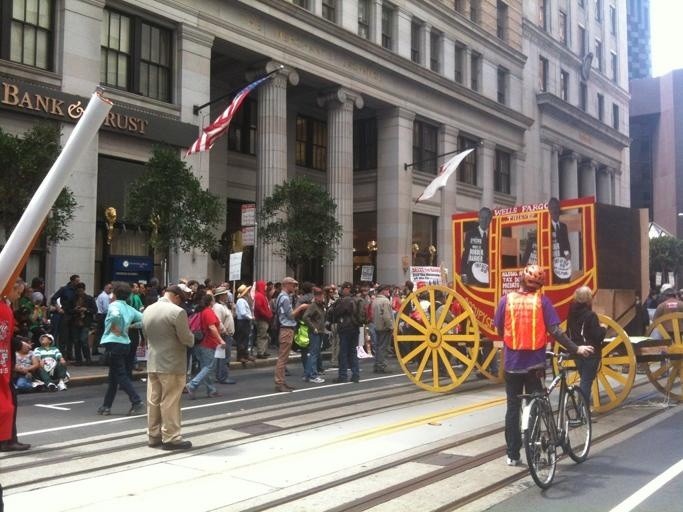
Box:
[[517, 350, 594, 488]]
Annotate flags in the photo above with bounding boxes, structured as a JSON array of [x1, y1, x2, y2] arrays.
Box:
[[182, 72, 275, 158], [414, 149, 473, 201]]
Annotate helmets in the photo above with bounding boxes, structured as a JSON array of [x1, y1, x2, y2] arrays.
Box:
[[523, 264, 544, 290]]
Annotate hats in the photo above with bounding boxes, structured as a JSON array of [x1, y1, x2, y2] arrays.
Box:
[[237, 284, 251, 298], [377, 284, 391, 294], [213, 287, 230, 297], [283, 276, 299, 283], [39, 333, 56, 346], [177, 283, 192, 292], [167, 284, 186, 303]]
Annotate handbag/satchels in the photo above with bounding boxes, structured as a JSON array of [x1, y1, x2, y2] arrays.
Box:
[[266, 314, 279, 339]]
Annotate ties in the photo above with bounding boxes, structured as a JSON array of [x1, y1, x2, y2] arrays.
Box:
[[555, 223, 563, 242], [482, 231, 486, 252]]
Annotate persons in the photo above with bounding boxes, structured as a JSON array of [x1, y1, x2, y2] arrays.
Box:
[[567, 286, 608, 425], [461, 207, 491, 289], [494, 265, 593, 466], [623, 285, 683, 337], [0, 274, 497, 453], [549, 195, 571, 285]]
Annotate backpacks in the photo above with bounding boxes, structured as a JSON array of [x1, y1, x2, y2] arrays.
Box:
[[188, 312, 205, 344], [343, 295, 369, 327], [294, 321, 309, 349]]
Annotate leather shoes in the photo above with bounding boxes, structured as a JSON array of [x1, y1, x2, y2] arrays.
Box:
[[162, 439, 192, 450], [221, 378, 237, 384], [275, 384, 293, 392], [351, 377, 360, 383], [284, 381, 298, 388], [0, 440, 31, 451], [333, 378, 347, 383]]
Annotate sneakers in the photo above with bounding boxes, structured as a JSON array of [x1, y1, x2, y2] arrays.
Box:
[[129, 402, 146, 414], [507, 457, 522, 466], [64, 351, 104, 367], [208, 390, 224, 398], [302, 369, 326, 383], [185, 384, 197, 401], [32, 379, 68, 392], [96, 405, 111, 416], [237, 352, 271, 362]]
